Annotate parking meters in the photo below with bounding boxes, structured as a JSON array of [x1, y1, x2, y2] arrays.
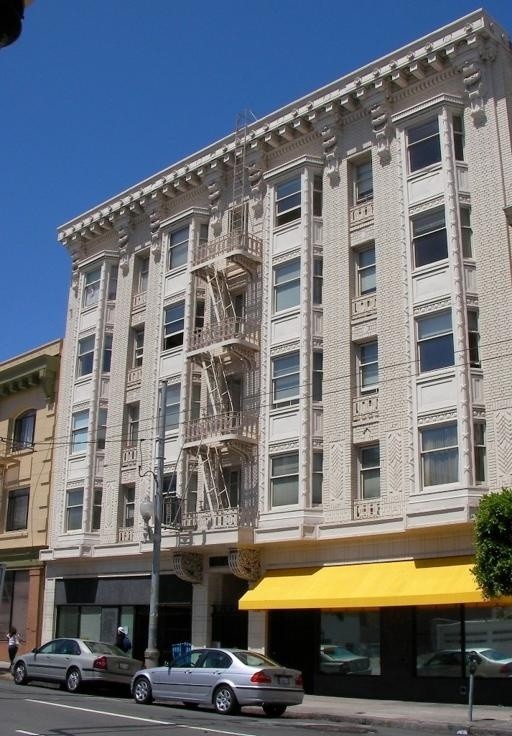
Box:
[[466, 651, 479, 721]]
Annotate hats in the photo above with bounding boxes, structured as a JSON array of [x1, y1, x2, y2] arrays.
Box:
[[118, 627, 128, 634]]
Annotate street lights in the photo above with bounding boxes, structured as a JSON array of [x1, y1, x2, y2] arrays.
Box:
[[140, 499, 164, 669]]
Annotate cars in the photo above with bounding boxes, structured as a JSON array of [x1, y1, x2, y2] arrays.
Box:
[[416, 647, 512, 678], [9, 638, 305, 719], [319, 644, 370, 675]]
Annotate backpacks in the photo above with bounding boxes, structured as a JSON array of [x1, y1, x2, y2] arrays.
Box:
[[121, 636, 131, 650]]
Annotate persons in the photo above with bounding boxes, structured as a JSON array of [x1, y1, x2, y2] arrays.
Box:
[[6, 626, 26, 669], [114, 627, 132, 654]]
